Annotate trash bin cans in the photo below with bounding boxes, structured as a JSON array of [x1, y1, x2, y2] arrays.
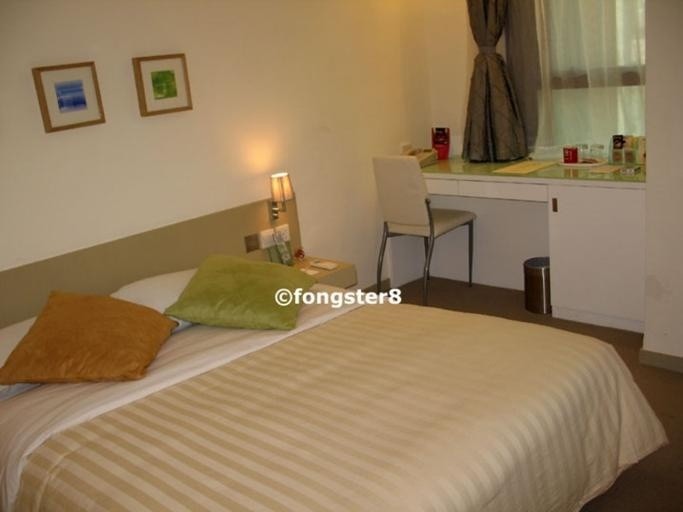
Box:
[[524, 258, 550, 314]]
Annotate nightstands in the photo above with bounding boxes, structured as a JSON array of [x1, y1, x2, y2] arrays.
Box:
[[286, 258, 357, 293]]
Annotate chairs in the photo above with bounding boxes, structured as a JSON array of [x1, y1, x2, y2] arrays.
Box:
[[372, 155, 478, 305]]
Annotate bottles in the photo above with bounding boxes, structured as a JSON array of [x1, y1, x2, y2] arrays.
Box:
[[559, 157, 608, 168]]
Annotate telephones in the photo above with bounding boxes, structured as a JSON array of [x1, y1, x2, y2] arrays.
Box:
[[401, 147, 438, 168]]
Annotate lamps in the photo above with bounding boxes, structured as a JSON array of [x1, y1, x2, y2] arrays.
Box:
[[267, 171, 293, 219]]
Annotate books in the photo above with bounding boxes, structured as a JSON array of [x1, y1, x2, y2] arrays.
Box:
[[589, 164, 625, 174], [489, 159, 560, 176]]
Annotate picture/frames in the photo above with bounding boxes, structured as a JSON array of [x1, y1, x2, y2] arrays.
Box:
[[31, 52, 193, 133]]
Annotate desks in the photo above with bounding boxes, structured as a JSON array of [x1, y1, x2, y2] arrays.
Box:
[[386, 159, 646, 336]]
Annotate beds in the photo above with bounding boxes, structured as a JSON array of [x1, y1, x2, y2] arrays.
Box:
[[0, 282, 619, 512]]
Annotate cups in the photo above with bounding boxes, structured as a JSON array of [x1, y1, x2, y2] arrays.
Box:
[[564, 146, 578, 163]]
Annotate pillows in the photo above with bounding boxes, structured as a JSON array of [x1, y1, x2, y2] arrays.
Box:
[[0, 255, 317, 400]]
